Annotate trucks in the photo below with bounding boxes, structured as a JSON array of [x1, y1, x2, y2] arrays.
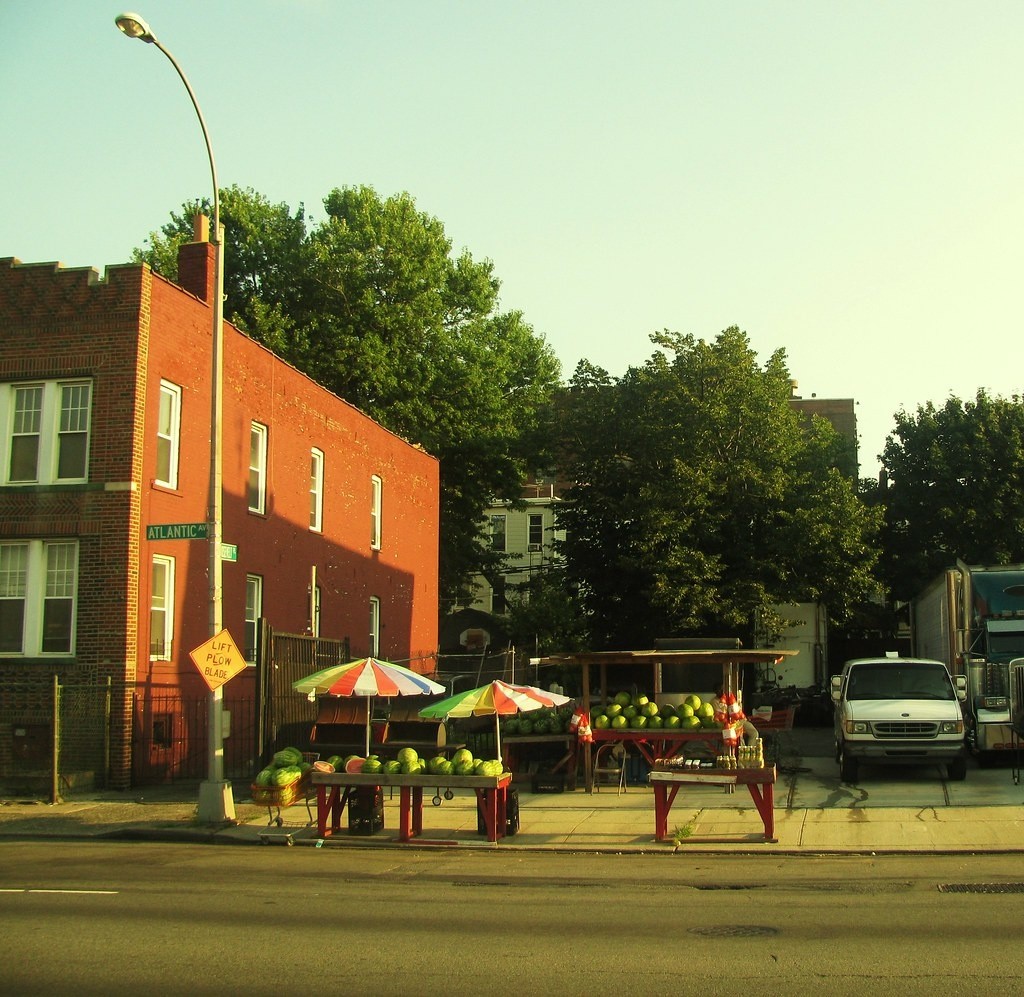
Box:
[[892, 557, 1024, 770]]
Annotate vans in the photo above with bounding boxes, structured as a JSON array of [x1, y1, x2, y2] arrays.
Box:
[[830, 657, 968, 783]]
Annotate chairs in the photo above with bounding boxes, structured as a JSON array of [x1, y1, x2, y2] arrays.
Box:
[[590, 744, 627, 796]]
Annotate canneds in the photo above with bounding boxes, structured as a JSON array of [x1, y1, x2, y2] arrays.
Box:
[[738, 737, 764, 768]]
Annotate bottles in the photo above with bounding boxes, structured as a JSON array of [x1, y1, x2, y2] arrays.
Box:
[[717, 756, 736, 771], [738, 738, 763, 767], [654, 756, 700, 769]]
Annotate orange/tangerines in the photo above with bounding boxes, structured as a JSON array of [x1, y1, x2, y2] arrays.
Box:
[[714, 698, 746, 747]]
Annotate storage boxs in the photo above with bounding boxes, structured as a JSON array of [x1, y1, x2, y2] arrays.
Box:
[[478, 787, 520, 837], [531, 773, 565, 793], [348, 788, 385, 836]]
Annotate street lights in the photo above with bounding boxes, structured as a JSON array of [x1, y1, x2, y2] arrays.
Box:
[[116, 9, 238, 826]]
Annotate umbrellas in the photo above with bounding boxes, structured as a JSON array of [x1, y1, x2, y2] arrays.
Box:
[[418, 678, 570, 762], [292, 657, 446, 758]]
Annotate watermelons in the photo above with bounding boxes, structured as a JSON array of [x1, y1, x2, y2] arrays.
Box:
[[590, 691, 724, 729], [502, 706, 575, 734], [254, 747, 313, 805], [313, 747, 504, 776]]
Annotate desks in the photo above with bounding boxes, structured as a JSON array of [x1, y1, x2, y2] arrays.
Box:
[[311, 741, 466, 800], [311, 772, 512, 843], [589, 728, 731, 782], [501, 733, 578, 792], [647, 759, 777, 842]]
